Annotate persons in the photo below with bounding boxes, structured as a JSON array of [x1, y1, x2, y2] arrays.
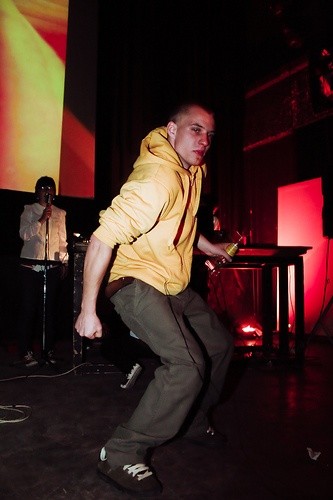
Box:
[[101, 311, 143, 390], [19, 176, 70, 368], [75, 102, 232, 497]]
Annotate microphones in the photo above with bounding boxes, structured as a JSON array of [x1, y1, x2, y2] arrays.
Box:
[[45, 193, 52, 206]]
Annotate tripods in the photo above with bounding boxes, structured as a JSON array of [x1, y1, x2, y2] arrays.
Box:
[[11, 207, 73, 370]]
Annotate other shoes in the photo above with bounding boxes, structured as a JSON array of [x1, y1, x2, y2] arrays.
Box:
[[120, 363, 142, 391], [48, 350, 57, 363], [97, 448, 164, 496], [201, 426, 226, 443], [21, 351, 39, 367]]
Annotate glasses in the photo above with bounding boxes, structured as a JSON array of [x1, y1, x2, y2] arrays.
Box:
[[38, 185, 54, 191]]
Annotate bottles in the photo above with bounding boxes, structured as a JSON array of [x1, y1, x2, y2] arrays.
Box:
[[213, 235, 244, 267]]
